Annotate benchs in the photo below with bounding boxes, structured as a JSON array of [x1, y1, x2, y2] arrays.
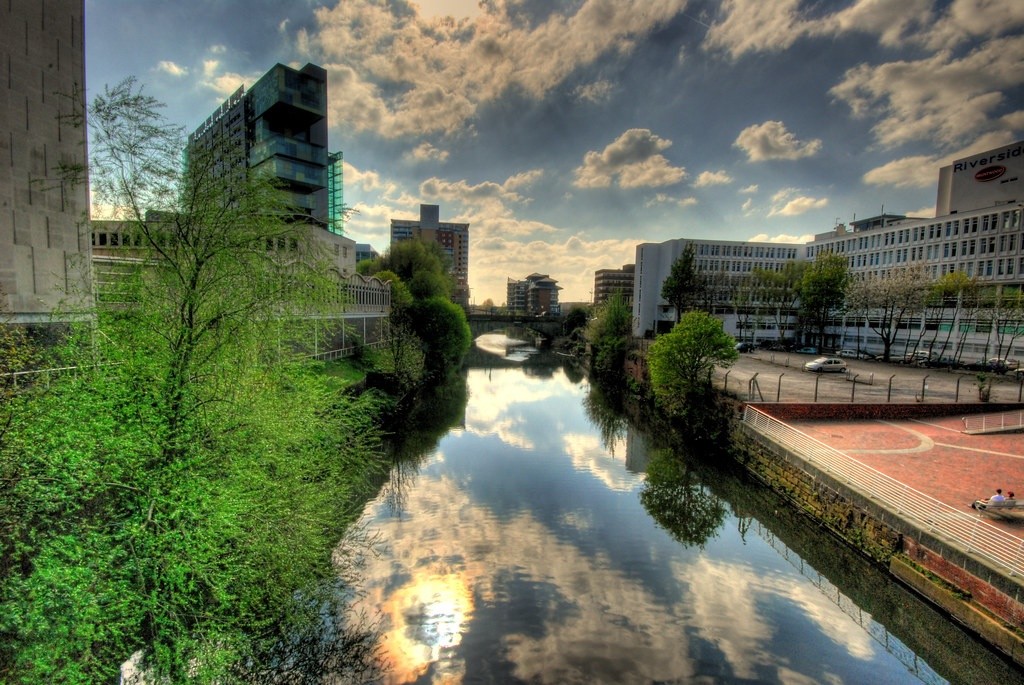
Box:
[[975, 498, 1024, 520]]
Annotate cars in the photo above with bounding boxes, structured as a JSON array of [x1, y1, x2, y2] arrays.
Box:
[[1014, 368, 1024, 377], [836, 349, 869, 360], [907, 351, 939, 361], [876, 354, 914, 364], [919, 355, 965, 370], [806, 357, 847, 373], [796, 347, 819, 355], [734, 342, 755, 353], [963, 360, 999, 372]]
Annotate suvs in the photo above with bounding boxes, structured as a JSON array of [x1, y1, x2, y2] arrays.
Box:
[[988, 357, 1019, 370]]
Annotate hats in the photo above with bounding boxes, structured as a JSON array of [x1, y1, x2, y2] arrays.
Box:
[[1007, 492, 1014, 497]]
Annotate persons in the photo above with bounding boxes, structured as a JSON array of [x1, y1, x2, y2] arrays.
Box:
[[966, 489, 1004, 511], [1003, 491, 1016, 500]]
